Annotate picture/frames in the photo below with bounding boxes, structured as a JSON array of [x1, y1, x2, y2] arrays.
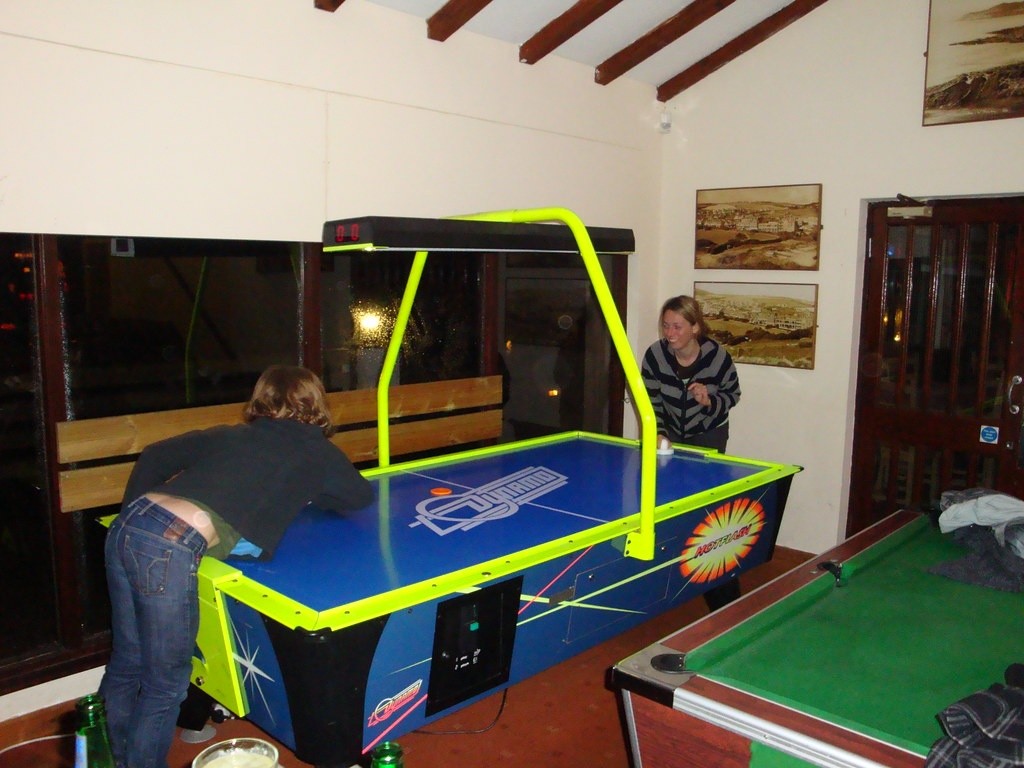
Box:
[[693, 182, 823, 271], [694, 281, 819, 370], [921, 0, 1024, 127]]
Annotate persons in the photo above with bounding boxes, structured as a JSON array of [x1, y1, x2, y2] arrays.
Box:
[[104, 363, 375, 768], [641, 296, 741, 454]]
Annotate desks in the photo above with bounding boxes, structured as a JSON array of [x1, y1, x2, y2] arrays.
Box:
[[604, 509, 1024, 768], [93, 429, 804, 768]]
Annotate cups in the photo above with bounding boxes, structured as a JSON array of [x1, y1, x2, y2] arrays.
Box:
[[191, 737, 280, 768]]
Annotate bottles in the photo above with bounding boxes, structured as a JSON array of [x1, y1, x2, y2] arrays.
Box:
[[369, 742, 406, 768], [71, 694, 116, 768]]
